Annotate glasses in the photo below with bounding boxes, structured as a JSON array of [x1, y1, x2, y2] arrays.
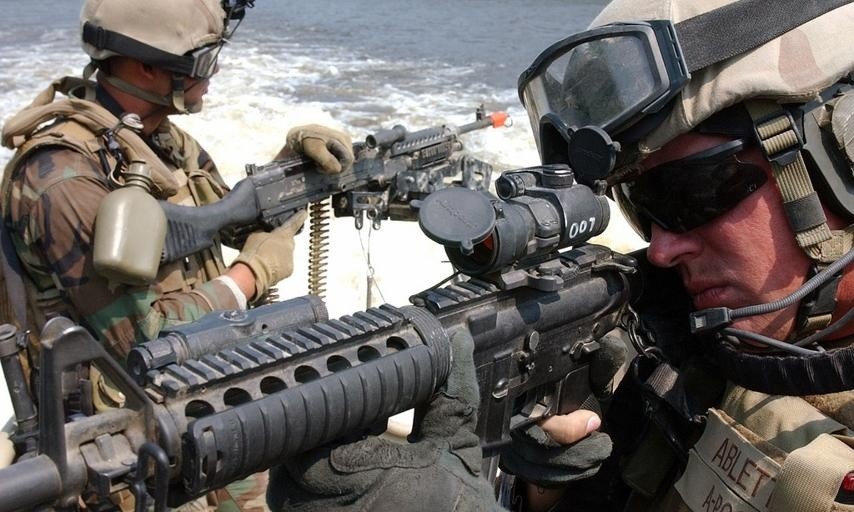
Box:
[[514, 3, 845, 161], [609, 136, 767, 242], [87, 27, 220, 80]]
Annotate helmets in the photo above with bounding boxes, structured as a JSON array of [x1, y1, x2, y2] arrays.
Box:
[[79, 0, 229, 60], [566, 3, 853, 179]]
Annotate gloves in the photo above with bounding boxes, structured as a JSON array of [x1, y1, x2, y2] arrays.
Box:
[[285, 126, 353, 174], [501, 414, 615, 486], [266, 329, 495, 511], [230, 209, 305, 305]]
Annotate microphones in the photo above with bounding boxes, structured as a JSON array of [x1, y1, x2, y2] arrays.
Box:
[[688, 246, 854, 339]]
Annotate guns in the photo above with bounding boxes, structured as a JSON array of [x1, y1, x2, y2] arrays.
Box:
[[0, 164, 682, 510], [163, 103, 511, 266]]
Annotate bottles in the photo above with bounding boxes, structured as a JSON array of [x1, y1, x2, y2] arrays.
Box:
[[91, 163, 167, 287]]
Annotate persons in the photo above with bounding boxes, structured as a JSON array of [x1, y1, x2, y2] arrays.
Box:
[[1, 0, 355, 511], [261, 0, 854, 512]]
